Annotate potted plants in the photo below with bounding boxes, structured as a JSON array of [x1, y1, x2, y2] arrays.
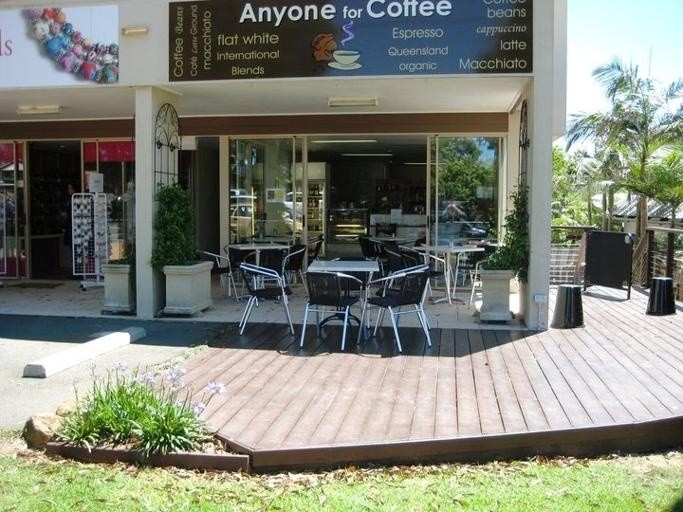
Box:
[[477, 181, 530, 324], [153, 182, 215, 317], [100, 254, 135, 316]]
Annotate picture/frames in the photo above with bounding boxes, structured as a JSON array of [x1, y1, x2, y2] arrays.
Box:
[[265, 188, 286, 202]]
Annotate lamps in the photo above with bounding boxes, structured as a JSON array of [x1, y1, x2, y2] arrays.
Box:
[[327, 96, 378, 107], [16, 105, 62, 116]]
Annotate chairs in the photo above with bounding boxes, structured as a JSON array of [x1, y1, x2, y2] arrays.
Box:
[[221, 231, 326, 276], [195, 249, 239, 304], [298, 269, 368, 353], [357, 261, 436, 354], [238, 261, 297, 337], [357, 232, 498, 310]]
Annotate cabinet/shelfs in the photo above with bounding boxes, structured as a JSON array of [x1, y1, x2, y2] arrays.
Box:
[[295, 180, 331, 257]]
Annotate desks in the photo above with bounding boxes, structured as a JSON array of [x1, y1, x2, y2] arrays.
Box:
[[302, 258, 381, 334]]
